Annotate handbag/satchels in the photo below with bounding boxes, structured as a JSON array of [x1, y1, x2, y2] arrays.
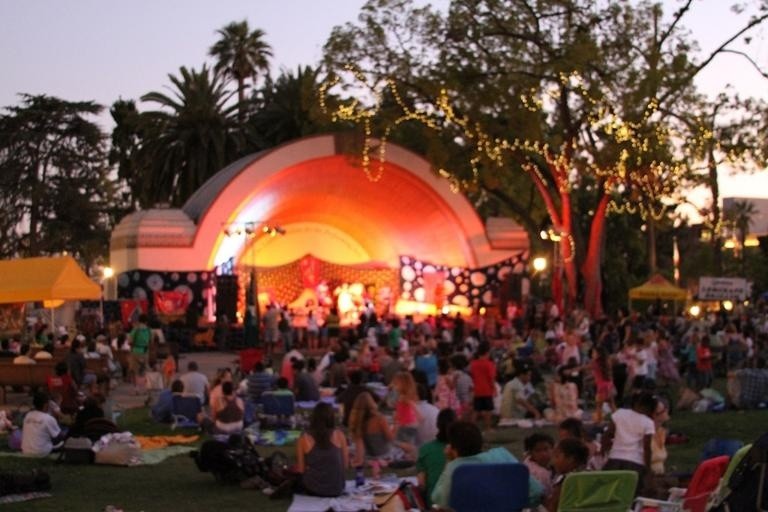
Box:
[[63, 435, 92, 463], [374, 483, 426, 512], [264, 451, 292, 486]]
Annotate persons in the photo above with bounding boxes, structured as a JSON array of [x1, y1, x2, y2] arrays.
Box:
[[1, 304, 164, 457], [164, 301, 768, 498], [432, 421, 544, 507], [539, 438, 589, 511]]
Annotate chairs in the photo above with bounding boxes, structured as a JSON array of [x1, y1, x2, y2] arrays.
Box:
[[447, 463, 530, 512], [635, 434, 767, 512], [558, 471, 638, 512]]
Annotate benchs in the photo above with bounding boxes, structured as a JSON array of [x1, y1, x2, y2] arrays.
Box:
[[0, 338, 187, 412]]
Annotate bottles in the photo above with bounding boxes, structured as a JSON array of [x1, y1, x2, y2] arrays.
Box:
[[355, 466, 365, 487]]
